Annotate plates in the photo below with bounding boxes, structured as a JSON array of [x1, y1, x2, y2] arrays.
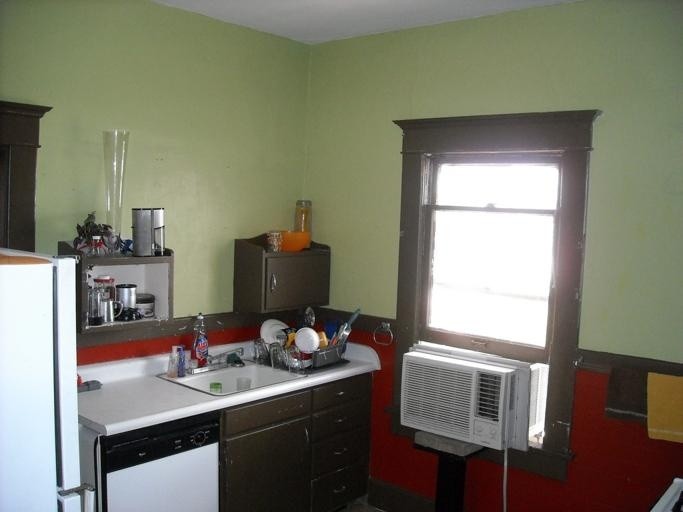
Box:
[[296, 327, 320, 354], [261, 319, 290, 348]]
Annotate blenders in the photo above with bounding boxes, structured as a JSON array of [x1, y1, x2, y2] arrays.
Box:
[[132, 208, 167, 257]]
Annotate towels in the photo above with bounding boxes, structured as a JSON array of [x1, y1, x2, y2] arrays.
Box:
[[603, 354, 683, 442]]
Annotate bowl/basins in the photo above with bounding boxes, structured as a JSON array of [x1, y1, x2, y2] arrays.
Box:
[[135, 293, 156, 314], [277, 231, 311, 252]]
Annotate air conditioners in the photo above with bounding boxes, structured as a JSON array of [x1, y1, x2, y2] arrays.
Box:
[[394, 341, 548, 456]]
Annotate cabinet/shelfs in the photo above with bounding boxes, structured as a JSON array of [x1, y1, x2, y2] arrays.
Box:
[[230, 234, 331, 316], [57, 237, 174, 348], [219, 375, 375, 512]]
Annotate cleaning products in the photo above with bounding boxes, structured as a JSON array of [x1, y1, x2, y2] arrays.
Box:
[[190, 311, 209, 368]]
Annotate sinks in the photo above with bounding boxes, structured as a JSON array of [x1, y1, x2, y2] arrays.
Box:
[[154, 358, 308, 397]]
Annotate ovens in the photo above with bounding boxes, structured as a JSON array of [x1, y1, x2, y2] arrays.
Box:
[[97, 425, 219, 511]]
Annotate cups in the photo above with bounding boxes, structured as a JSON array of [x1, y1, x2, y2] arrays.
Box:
[[102, 129, 129, 254], [251, 338, 306, 375], [236, 377, 251, 389], [209, 382, 222, 394]]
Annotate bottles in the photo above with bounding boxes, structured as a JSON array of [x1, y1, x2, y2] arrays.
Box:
[[295, 199, 312, 249], [89, 275, 135, 322], [85, 236, 109, 258], [168, 344, 186, 379], [267, 232, 280, 254]]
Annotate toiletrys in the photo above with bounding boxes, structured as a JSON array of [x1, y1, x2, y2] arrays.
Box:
[[178, 344, 186, 377], [168, 345, 180, 378]]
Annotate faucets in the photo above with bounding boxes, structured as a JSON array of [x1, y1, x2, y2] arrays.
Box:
[[207, 346, 246, 366]]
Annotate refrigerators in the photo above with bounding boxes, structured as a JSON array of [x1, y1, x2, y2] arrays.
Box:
[[0, 243, 94, 511]]
